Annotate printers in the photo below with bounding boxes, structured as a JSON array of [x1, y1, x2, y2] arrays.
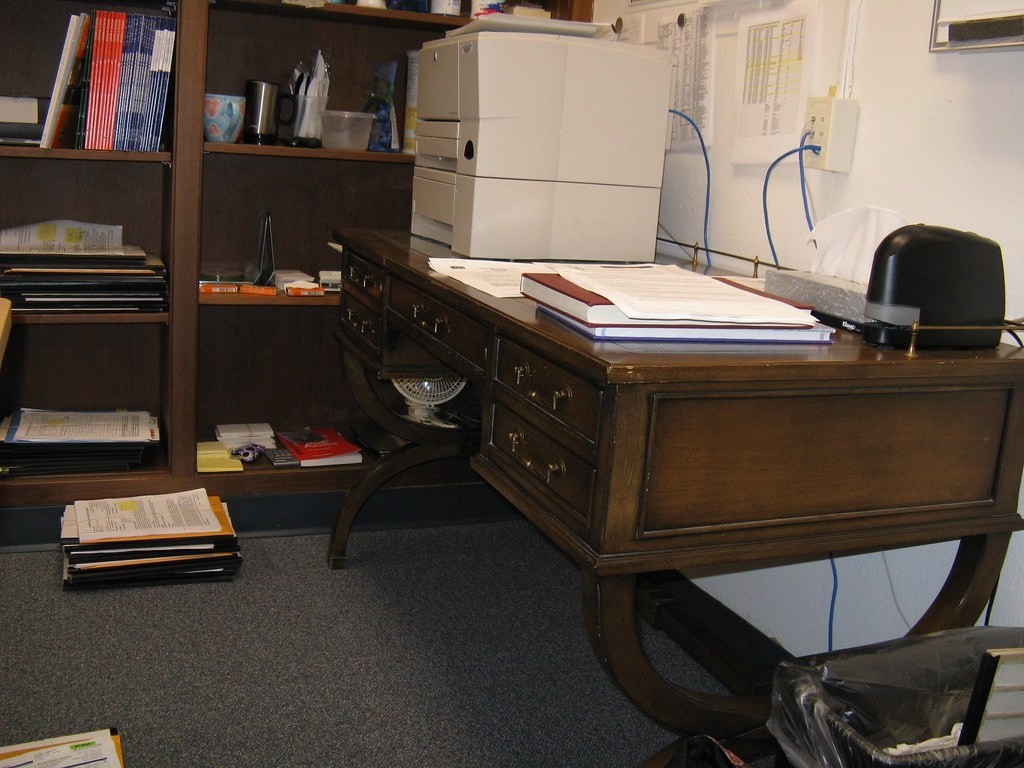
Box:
[[410, 30, 681, 263]]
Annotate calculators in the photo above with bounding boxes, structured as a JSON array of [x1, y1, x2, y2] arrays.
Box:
[[264, 449, 299, 469]]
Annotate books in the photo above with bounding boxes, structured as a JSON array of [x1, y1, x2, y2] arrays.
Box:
[[956, 648, 1024, 750], [519, 273, 835, 345], [0, 9, 362, 768]]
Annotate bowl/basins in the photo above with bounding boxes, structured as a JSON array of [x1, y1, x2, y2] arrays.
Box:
[[319, 110, 374, 150]]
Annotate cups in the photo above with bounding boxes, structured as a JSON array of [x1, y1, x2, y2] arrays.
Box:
[[291, 95, 329, 148], [244, 79, 297, 144], [205, 93, 246, 143]]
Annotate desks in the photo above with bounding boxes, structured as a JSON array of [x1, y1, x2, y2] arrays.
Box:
[[323, 227, 1024, 768]]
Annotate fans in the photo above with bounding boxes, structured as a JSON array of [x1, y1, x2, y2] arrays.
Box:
[[389, 377, 467, 428]]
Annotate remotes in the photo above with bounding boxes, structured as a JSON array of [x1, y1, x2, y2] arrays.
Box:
[[265, 448, 299, 467]]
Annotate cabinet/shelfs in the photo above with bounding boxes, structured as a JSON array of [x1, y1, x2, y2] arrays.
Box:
[[0, 0, 594, 551]]
